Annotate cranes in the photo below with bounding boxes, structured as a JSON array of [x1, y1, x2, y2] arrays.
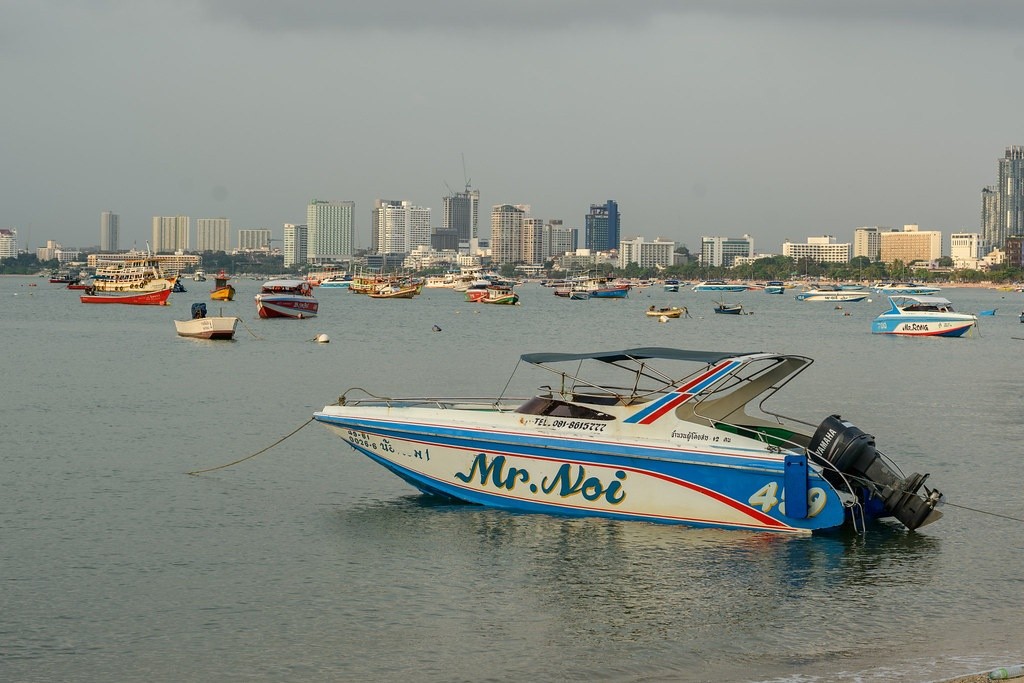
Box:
[[465, 177, 472, 193]]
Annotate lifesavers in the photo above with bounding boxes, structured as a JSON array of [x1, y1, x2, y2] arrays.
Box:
[[92, 285, 104, 291], [115, 287, 123, 291], [130, 281, 147, 289]]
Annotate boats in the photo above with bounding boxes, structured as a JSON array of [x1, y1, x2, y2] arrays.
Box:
[[713, 305, 742, 314], [301, 260, 787, 307], [39, 255, 189, 307], [311, 348, 954, 545], [209, 266, 235, 302], [794, 280, 942, 302], [871, 294, 979, 339], [979, 307, 998, 316], [174, 307, 239, 340], [254, 279, 320, 319], [646, 307, 691, 319], [192, 266, 207, 282]]
